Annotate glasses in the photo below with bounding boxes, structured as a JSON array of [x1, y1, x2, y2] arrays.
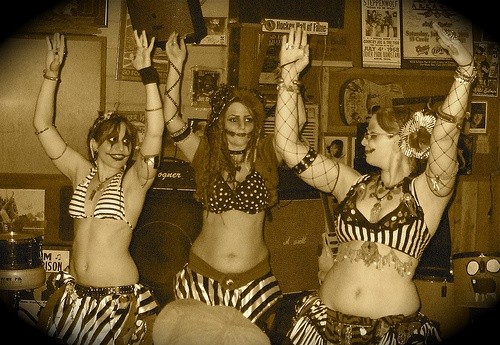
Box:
[[365, 127, 395, 140]]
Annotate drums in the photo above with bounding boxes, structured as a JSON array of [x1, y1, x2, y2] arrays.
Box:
[[452, 251, 500, 309], [0, 232, 44, 269]]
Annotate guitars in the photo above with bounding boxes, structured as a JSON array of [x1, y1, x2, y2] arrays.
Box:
[[338, 78, 448, 127]]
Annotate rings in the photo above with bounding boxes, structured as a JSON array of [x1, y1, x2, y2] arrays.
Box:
[[304, 54, 309, 57], [64, 53, 65, 54], [130, 56, 135, 62], [295, 47, 298, 49], [174, 42, 176, 43], [443, 28, 458, 39], [143, 46, 145, 48]]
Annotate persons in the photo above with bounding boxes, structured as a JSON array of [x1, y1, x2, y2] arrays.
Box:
[[33, 29, 165, 345], [163, 32, 308, 345], [367, 10, 392, 33], [274, 20, 476, 345]]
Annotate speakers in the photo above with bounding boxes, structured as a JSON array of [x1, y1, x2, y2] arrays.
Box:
[[120, 162, 203, 306], [262, 170, 340, 293], [126, 0, 208, 49]]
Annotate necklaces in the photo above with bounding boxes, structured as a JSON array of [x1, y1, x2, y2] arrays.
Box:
[[227, 150, 247, 172], [89, 170, 120, 200], [370, 171, 418, 223]]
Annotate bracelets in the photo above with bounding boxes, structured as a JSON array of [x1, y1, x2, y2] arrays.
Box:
[[138, 66, 160, 86], [276, 78, 303, 87], [277, 85, 300, 93], [45, 76, 58, 81], [145, 107, 163, 111], [453, 69, 477, 85]]
